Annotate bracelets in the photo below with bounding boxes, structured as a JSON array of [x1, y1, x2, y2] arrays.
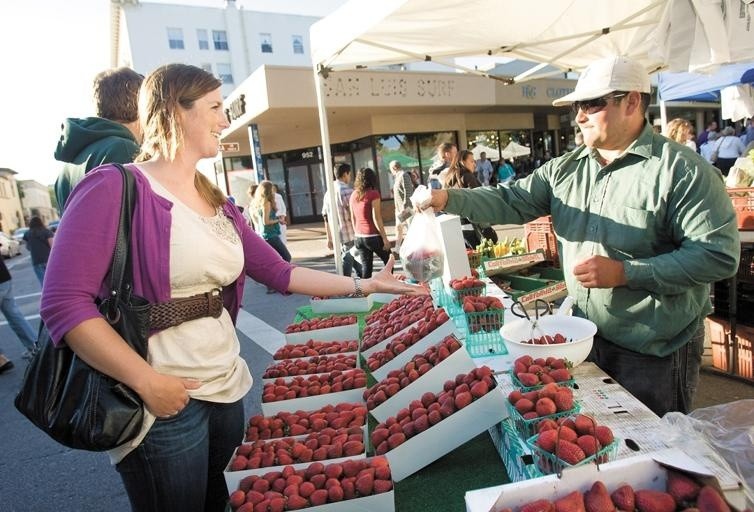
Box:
[[353, 276, 364, 298]]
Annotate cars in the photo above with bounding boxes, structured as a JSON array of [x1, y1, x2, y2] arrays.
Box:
[[0, 229, 23, 259], [46, 220, 59, 232], [11, 227, 30, 243]]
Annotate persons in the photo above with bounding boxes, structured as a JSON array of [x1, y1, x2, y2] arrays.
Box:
[[0, 246, 38, 374], [222, 180, 292, 298], [54, 67, 146, 217], [653, 114, 754, 178], [39, 64, 430, 512], [321, 140, 574, 280], [22, 217, 53, 287], [410, 53, 742, 419]]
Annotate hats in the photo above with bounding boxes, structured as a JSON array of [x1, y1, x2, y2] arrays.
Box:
[[552, 55, 651, 108]]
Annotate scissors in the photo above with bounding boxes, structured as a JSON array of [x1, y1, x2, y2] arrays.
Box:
[[511, 299, 550, 344]]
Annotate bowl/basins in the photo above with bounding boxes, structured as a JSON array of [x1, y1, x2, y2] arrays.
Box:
[[497, 315, 599, 367]]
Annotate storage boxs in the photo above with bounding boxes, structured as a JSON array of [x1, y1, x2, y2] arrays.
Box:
[[221, 272, 731, 511]]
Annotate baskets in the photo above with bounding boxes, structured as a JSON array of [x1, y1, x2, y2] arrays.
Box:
[[726, 187, 754, 231], [505, 368, 619, 477], [524, 214, 558, 260], [437, 274, 508, 359], [468, 250, 481, 269]]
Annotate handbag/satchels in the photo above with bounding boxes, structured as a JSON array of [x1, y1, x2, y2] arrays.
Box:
[[14, 293, 151, 452]]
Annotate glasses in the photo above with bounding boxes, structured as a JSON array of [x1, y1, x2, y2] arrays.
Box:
[[570, 92, 630, 116]]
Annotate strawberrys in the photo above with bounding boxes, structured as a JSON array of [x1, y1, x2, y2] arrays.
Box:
[[520, 334, 566, 344], [509, 355, 615, 473], [463, 295, 505, 332], [449, 275, 483, 302], [229, 316, 394, 512], [361, 294, 494, 453], [494, 477, 732, 512], [312, 294, 369, 300]]
